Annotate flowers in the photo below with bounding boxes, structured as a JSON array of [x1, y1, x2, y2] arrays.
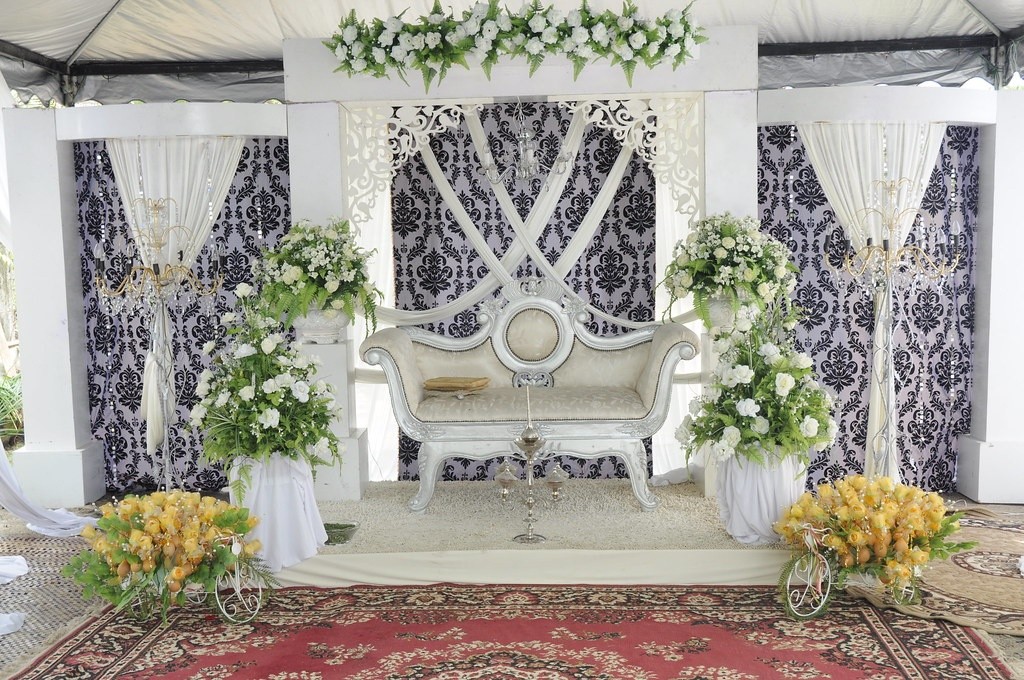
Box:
[[189, 282, 341, 505], [665, 212, 798, 329], [325, 0, 706, 87], [774, 474, 979, 606], [73, 490, 281, 625], [257, 219, 384, 340], [675, 342, 837, 464]]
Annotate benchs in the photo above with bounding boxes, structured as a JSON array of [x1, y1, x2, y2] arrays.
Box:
[[359, 275, 699, 512]]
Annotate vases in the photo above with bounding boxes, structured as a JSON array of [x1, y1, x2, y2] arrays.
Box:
[[289, 304, 351, 345], [704, 286, 748, 329]]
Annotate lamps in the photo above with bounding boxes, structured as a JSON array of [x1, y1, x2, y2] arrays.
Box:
[[485, 98, 572, 186], [821, 173, 960, 479], [91, 195, 225, 493]]
